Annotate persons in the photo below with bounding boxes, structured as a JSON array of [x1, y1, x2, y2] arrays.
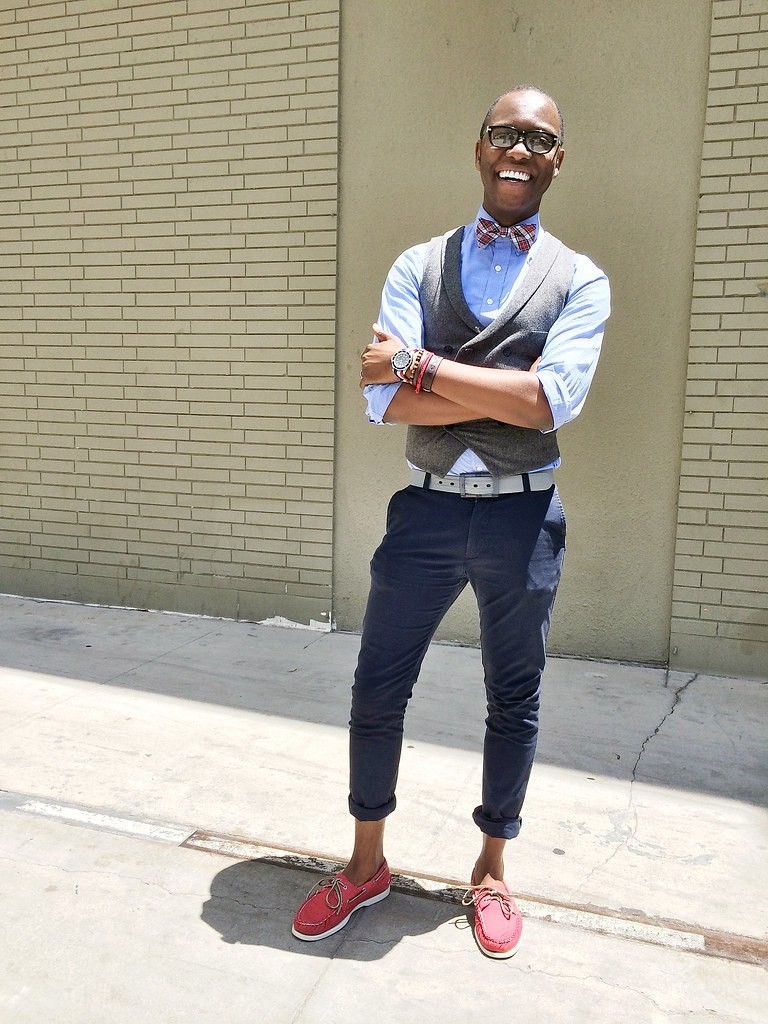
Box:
[[292, 86, 612, 961]]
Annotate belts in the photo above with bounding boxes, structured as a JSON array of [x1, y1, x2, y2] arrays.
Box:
[[409, 469, 555, 497]]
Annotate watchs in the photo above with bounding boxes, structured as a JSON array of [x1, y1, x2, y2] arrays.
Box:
[[390, 347, 415, 383]]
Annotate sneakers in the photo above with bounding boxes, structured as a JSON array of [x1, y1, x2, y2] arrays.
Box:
[[291, 857, 390, 942], [469, 873, 523, 959]]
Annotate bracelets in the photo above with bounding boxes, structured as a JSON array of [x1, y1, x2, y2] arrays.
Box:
[[409, 341, 443, 397]]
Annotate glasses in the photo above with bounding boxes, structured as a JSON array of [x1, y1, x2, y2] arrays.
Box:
[[481, 124, 563, 154]]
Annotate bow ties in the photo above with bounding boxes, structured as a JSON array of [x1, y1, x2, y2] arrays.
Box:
[[476, 217, 536, 253]]
